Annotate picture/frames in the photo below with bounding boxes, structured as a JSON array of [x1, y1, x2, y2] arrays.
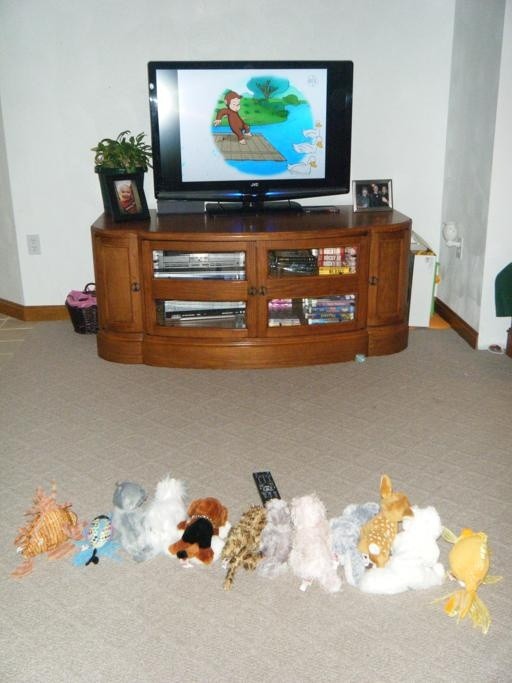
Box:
[[352, 178, 394, 213], [106, 174, 151, 221]]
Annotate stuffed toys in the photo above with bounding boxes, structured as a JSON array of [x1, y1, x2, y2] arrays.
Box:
[[220, 493, 340, 592], [12, 484, 80, 574], [70, 512, 120, 566], [110, 476, 232, 566], [441, 528, 502, 629], [10, 472, 504, 633], [329, 474, 457, 592]]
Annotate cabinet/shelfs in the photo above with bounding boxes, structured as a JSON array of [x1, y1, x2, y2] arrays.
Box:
[[91, 204, 412, 370]]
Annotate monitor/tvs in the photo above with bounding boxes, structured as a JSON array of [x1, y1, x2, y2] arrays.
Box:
[[147, 59, 354, 215]]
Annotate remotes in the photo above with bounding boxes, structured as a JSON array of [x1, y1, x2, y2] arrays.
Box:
[[252, 470, 281, 508]]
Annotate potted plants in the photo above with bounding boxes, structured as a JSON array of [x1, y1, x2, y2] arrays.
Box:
[[90, 130, 153, 213]]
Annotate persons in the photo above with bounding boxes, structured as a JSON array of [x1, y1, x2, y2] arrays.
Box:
[[356, 183, 389, 209], [117, 184, 139, 214]]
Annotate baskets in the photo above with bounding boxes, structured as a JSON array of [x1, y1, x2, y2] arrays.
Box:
[[63, 283, 99, 335]]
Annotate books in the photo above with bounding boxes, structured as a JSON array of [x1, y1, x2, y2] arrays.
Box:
[[266, 246, 358, 327]]
